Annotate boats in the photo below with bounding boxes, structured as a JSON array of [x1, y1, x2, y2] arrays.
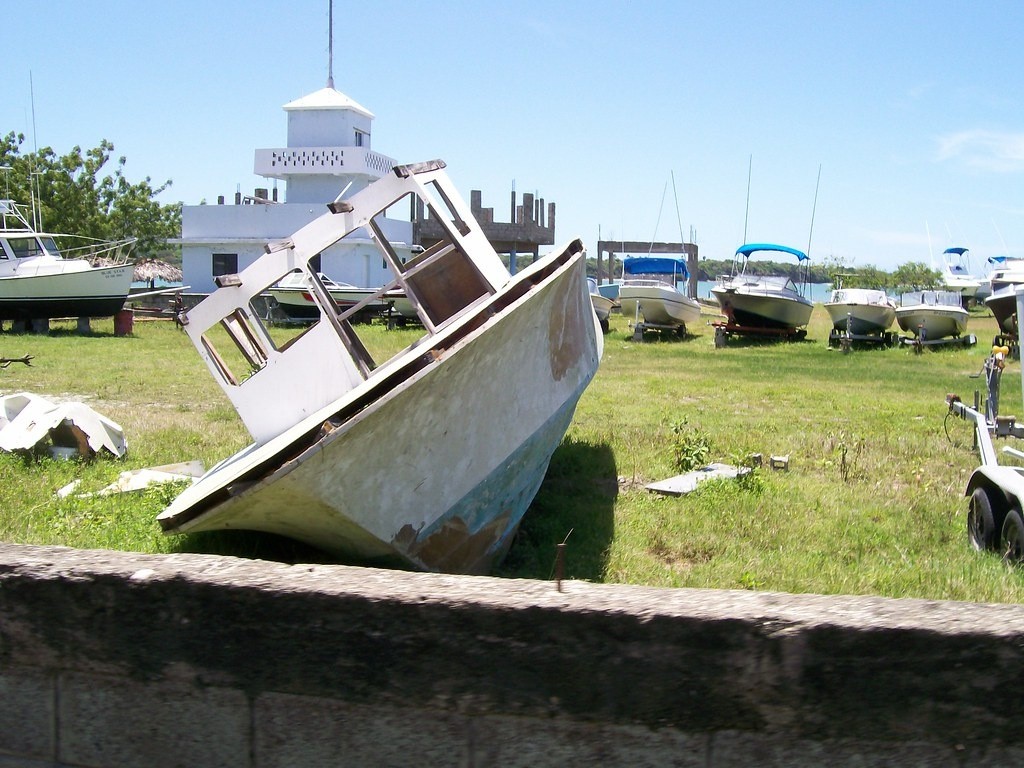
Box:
[[267, 271, 389, 319], [0, 198, 139, 320], [157, 160, 608, 575], [382, 285, 418, 319], [895, 290, 969, 342], [619, 256, 702, 326], [823, 272, 895, 334], [984, 254, 1024, 336], [587, 276, 616, 323], [943, 246, 981, 298], [710, 242, 815, 329]]
